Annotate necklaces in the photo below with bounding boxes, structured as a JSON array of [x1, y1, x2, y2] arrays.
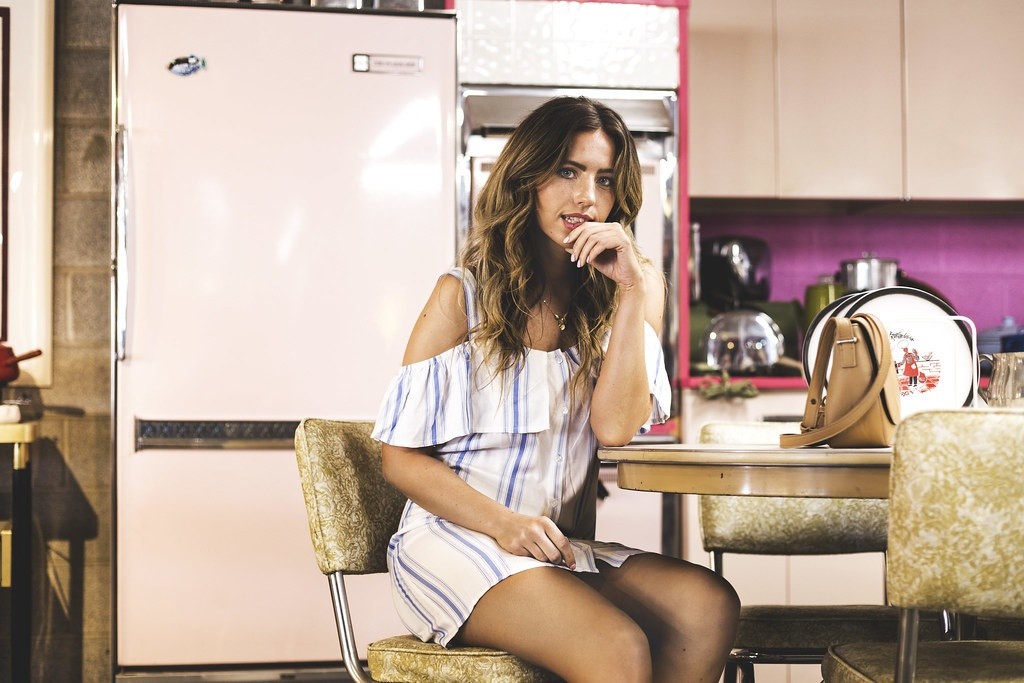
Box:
[[540, 297, 573, 331]]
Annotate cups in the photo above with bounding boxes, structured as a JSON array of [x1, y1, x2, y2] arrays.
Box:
[[979, 352, 1024, 407], [0, 405, 20, 423]]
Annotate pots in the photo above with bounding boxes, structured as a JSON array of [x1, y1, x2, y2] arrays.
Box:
[[834, 253, 900, 294]]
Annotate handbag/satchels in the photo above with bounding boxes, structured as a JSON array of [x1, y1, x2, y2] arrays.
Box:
[[780, 312, 900, 449]]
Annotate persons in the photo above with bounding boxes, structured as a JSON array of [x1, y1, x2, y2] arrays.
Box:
[[364, 98, 741, 683]]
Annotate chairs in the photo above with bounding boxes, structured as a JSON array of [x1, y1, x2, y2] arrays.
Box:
[[699, 407, 1024, 683], [294, 419, 559, 683]]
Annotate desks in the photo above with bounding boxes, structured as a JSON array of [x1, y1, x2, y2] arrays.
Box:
[[595, 445, 895, 591], [0, 418, 38, 683]]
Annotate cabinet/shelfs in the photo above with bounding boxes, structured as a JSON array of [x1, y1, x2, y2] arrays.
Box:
[[687, 0, 1024, 213]]
